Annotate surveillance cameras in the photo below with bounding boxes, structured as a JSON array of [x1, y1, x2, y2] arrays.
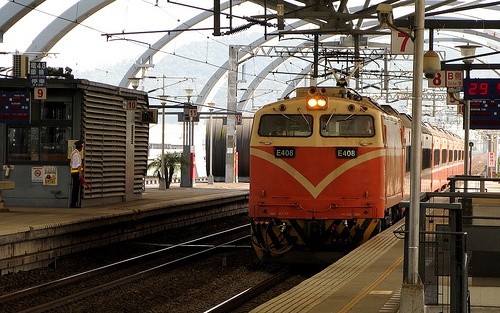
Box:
[[376, 4, 394, 29]]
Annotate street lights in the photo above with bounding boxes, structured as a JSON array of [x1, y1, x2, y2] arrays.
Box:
[[454, 41, 483, 192], [156, 94, 171, 190], [183, 88, 193, 145], [206, 101, 217, 184]]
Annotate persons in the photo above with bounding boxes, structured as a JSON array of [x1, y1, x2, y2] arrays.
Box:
[[357, 118, 373, 135], [269, 119, 286, 135], [69, 141, 84, 208]]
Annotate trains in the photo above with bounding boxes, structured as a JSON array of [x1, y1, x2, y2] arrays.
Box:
[[248, 86, 470, 264]]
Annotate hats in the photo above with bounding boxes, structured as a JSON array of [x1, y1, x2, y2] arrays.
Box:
[[76, 144, 83, 148]]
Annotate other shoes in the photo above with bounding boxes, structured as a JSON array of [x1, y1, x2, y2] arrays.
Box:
[[70, 204, 81, 208]]
